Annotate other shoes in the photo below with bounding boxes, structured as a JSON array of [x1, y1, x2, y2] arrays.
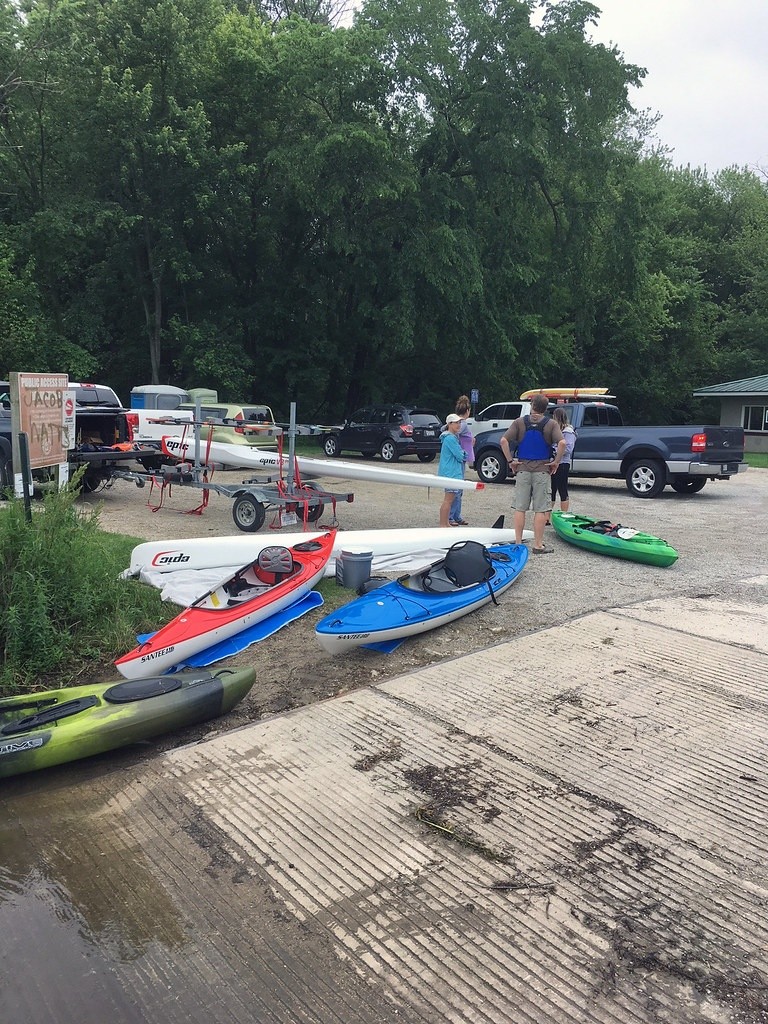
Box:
[[545, 520, 551, 526]]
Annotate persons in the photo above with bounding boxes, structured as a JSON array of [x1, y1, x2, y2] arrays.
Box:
[[547, 409, 575, 525], [437, 394, 475, 526], [501, 393, 568, 553]]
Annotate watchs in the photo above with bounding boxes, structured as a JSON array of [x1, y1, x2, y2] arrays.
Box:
[[507, 459, 514, 463]]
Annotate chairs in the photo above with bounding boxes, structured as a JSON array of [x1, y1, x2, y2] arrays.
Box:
[[231, 545, 293, 601], [424, 541, 492, 592]]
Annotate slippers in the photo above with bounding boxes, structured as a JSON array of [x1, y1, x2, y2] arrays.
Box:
[[532, 544, 554, 554]]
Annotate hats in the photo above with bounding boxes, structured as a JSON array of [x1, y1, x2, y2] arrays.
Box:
[[446, 414, 464, 424]]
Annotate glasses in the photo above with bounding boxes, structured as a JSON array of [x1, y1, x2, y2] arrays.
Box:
[[530, 403, 532, 406]]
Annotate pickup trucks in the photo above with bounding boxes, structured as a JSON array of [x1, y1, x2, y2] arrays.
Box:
[[468, 401, 749, 499]]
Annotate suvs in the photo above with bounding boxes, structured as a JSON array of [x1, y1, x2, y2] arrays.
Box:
[[0, 384, 281, 502], [320, 401, 444, 464], [464, 401, 556, 441]]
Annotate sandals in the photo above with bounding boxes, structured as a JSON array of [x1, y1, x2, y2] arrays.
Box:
[[457, 521, 468, 525], [449, 521, 459, 526]]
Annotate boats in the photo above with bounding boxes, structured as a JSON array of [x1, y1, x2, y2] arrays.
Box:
[[114, 527, 337, 679], [551, 508, 679, 568], [315, 539, 529, 656], [0, 665, 257, 780]]
